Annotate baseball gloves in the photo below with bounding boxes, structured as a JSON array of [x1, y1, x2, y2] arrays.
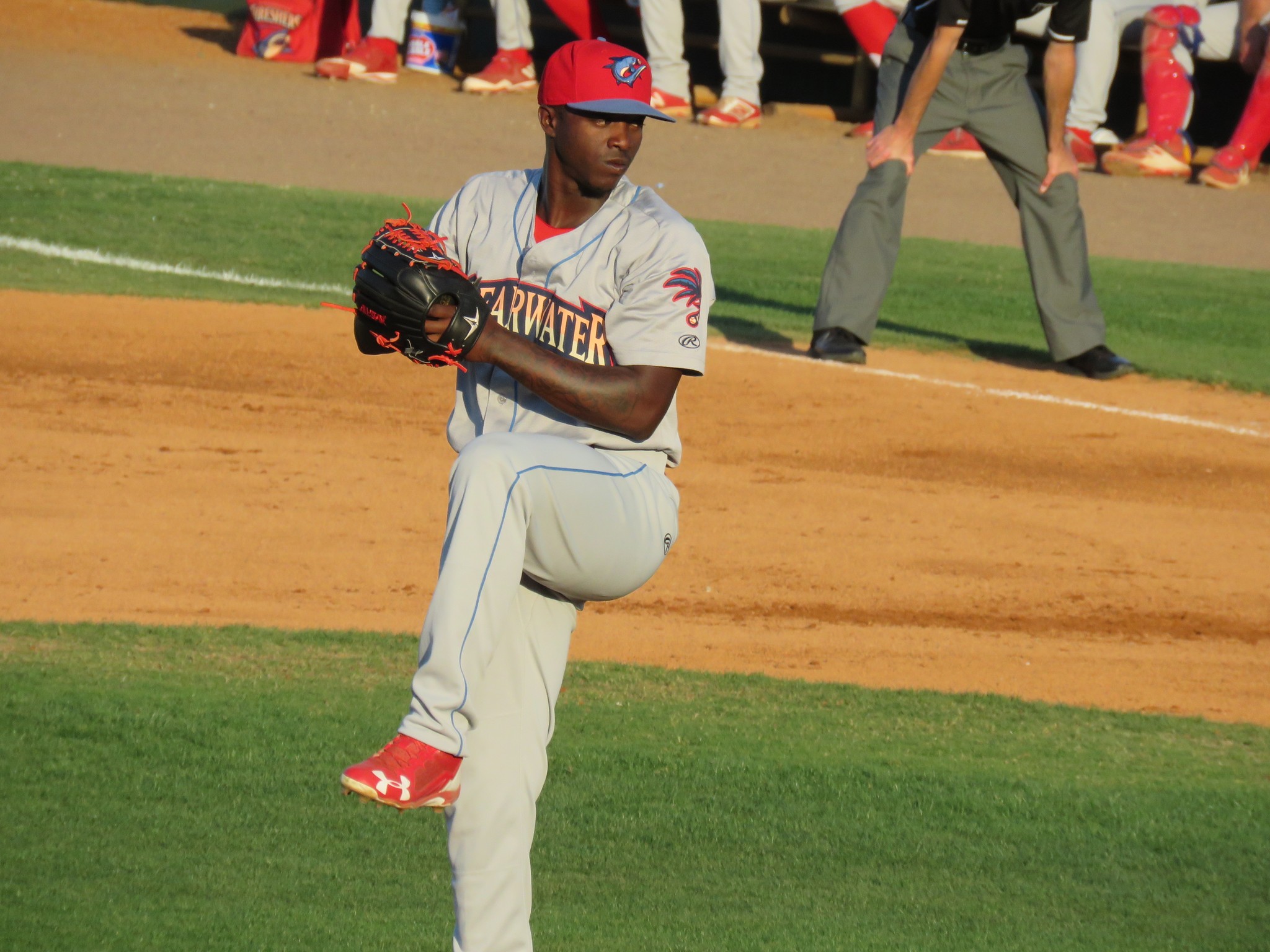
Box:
[[353, 218, 491, 368]]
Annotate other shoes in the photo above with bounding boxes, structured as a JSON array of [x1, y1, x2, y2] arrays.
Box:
[[928, 128, 987, 160]]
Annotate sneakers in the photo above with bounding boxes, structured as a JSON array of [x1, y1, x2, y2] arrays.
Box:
[[846, 122, 873, 139], [460, 48, 536, 92], [810, 328, 866, 365], [1069, 345, 1132, 377], [644, 83, 694, 119], [340, 734, 461, 813], [1055, 126, 1097, 170], [698, 91, 763, 131], [1100, 131, 1191, 183], [315, 35, 398, 86], [1197, 147, 1252, 190]]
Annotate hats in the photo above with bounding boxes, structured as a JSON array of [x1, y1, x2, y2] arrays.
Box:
[[538, 35, 677, 124]]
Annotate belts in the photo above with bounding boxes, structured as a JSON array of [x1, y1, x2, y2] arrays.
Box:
[[958, 39, 1001, 57]]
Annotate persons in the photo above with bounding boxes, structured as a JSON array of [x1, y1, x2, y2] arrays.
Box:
[[807, 0, 1136, 382], [314, 0, 540, 93], [637, 0, 765, 128], [833, 0, 1270, 190], [343, 37, 717, 952]]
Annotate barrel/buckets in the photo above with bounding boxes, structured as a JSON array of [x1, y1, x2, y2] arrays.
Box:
[[403, 8, 468, 75]]
